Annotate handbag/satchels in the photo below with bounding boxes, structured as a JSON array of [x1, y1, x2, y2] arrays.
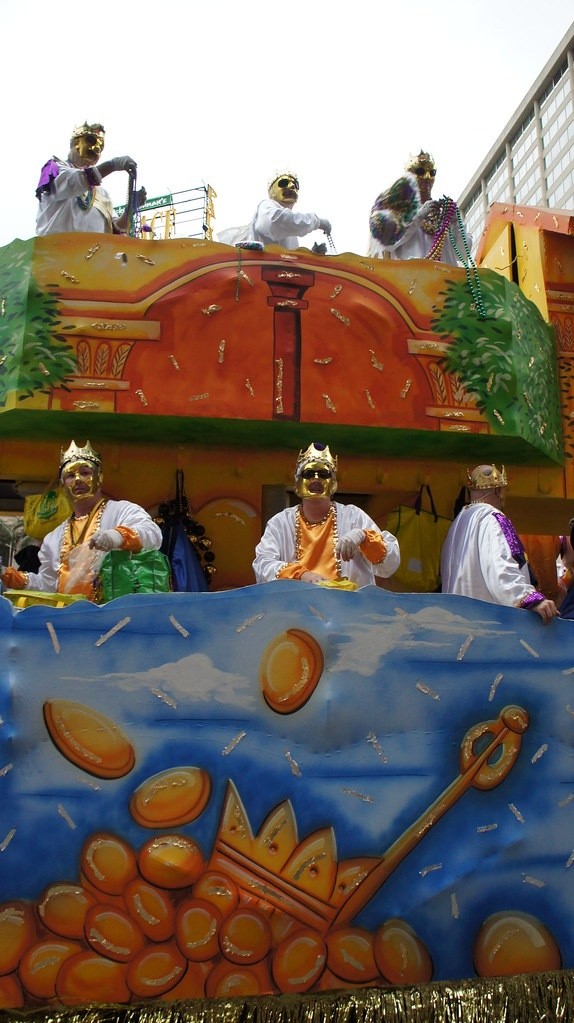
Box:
[[101, 549, 171, 605]]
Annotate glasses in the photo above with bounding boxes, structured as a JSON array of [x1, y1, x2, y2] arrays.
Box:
[[301, 469, 332, 480], [415, 167, 436, 176]]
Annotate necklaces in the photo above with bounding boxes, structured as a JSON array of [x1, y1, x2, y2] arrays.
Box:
[[70, 513, 90, 545], [67, 158, 97, 212], [326, 232, 338, 254], [423, 194, 488, 322], [124, 167, 144, 238]]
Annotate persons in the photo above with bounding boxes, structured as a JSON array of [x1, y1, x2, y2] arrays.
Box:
[[368, 148, 472, 267], [0, 440, 162, 606], [251, 443, 400, 591], [35, 121, 147, 236], [253, 169, 331, 254], [441, 464, 561, 625]]
